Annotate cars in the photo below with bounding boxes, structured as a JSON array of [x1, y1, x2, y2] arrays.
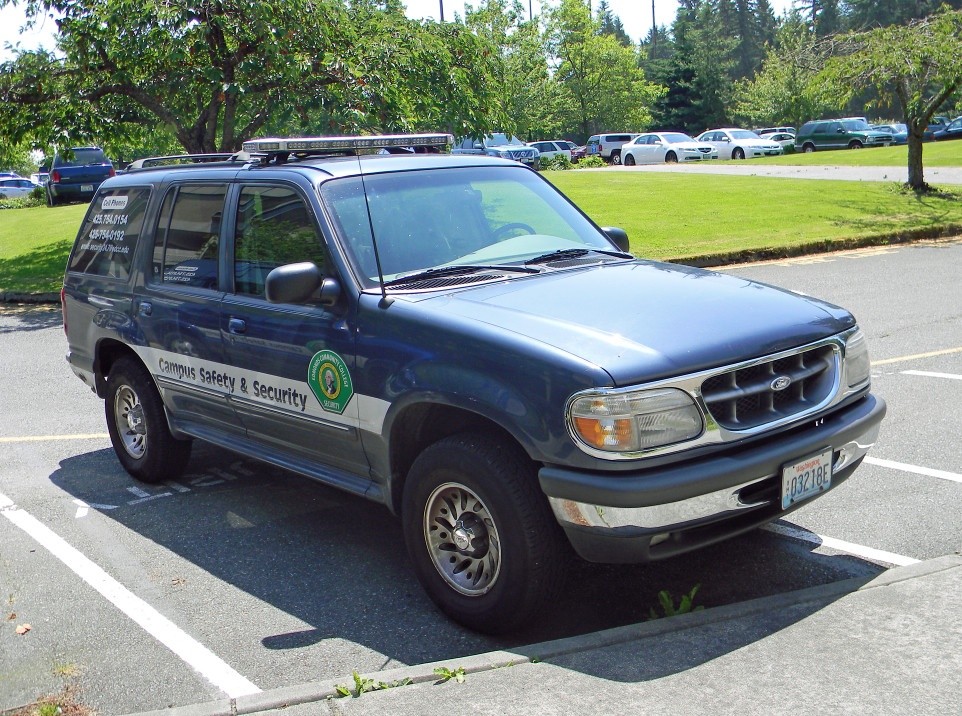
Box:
[[842, 116, 962, 145], [451, 131, 539, 170], [693, 128, 784, 160], [761, 133, 795, 148], [526, 139, 586, 166], [620, 132, 720, 166]]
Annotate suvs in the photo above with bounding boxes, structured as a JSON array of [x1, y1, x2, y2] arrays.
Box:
[[794, 120, 897, 153], [59, 133, 886, 635], [39, 147, 117, 206], [0, 174, 42, 199]]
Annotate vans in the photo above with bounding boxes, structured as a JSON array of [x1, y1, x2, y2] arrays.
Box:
[[587, 133, 655, 165], [753, 127, 796, 139]]
